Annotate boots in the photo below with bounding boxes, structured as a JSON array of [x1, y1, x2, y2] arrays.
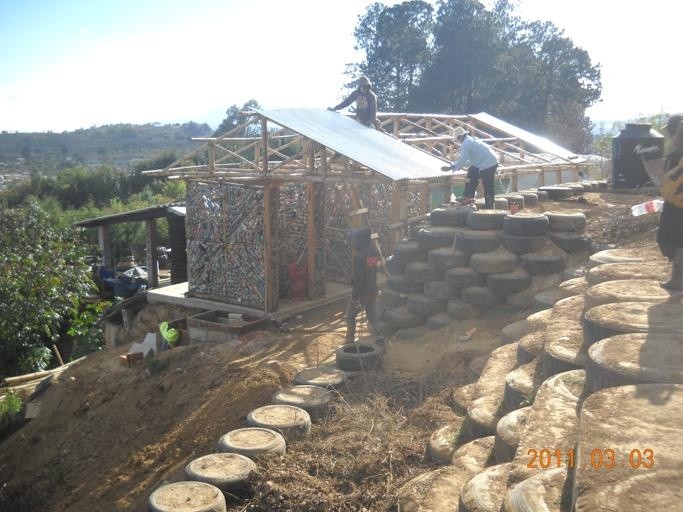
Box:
[[660, 249, 683, 290]]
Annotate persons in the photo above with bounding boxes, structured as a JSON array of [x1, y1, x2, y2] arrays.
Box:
[[327, 76, 382, 130], [441, 126, 499, 209], [344, 224, 394, 349], [654, 114, 683, 292]]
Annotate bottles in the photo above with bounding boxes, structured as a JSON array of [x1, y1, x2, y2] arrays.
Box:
[[630, 199, 664, 217]]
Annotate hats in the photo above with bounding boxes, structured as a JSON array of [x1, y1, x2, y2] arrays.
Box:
[[451, 126, 470, 142], [660, 112, 683, 131]]
